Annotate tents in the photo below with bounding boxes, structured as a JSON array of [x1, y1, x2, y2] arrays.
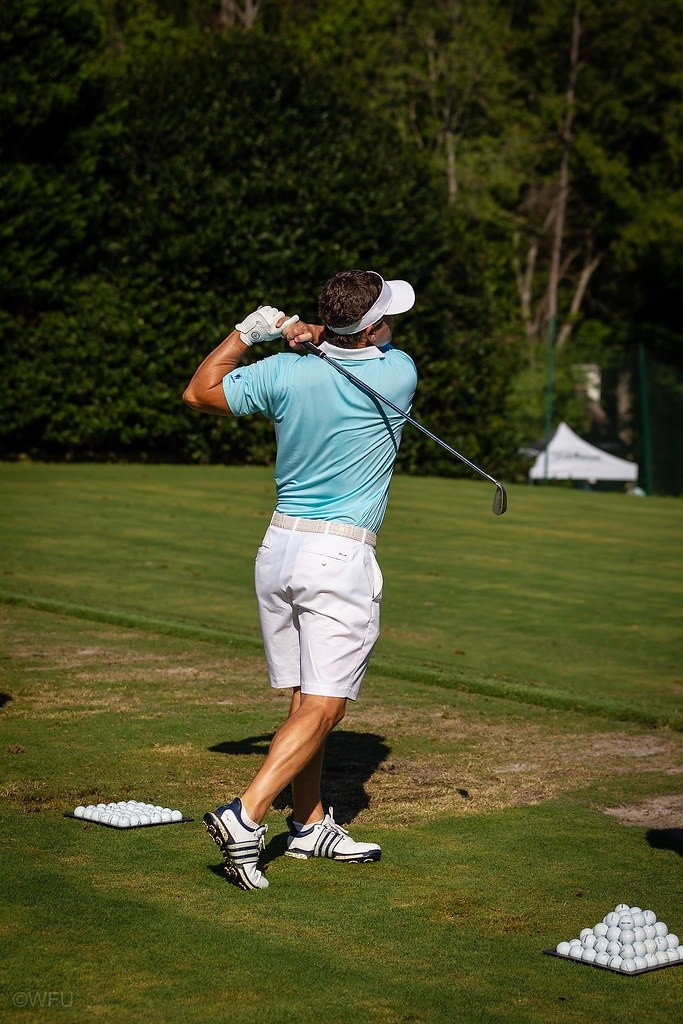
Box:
[[522, 419, 639, 490]]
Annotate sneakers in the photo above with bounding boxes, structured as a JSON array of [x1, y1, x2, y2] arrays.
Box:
[[203, 796, 270, 890], [283, 805, 381, 864]]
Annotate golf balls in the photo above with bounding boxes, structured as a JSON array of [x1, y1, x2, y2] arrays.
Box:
[[73, 799, 182, 827], [557, 902, 683, 972]]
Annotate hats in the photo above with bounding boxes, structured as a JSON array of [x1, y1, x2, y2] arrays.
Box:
[[324, 271, 417, 336]]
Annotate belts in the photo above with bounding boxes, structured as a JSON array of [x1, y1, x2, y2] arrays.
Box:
[[271, 514, 377, 547]]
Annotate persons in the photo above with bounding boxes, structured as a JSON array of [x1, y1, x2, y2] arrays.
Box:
[[183, 269, 417, 887]]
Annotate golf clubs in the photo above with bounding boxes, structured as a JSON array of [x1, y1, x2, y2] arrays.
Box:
[[302, 340, 509, 516]]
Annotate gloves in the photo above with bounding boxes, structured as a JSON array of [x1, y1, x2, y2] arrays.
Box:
[[235, 306, 300, 346]]
